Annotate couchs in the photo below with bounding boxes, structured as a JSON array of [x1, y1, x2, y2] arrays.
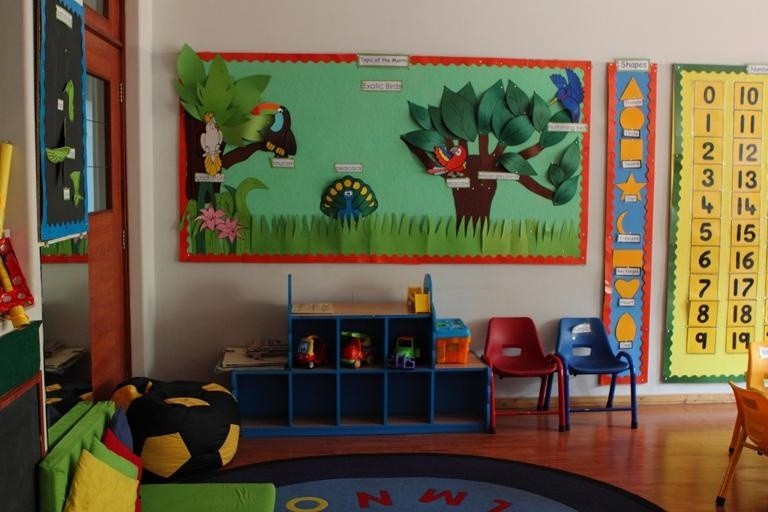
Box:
[[40, 401, 277, 512]]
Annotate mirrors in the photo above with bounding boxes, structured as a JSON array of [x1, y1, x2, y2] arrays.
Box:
[[40, 235, 94, 452]]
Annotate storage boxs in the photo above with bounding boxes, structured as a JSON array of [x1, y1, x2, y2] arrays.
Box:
[[0, 319, 44, 395]]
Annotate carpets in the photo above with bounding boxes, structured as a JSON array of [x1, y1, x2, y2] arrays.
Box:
[[192, 451, 624, 512]]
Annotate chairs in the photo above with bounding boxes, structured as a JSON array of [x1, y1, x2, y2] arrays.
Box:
[[483, 315, 565, 434], [715, 380, 768, 504], [730, 340, 768, 456], [543, 315, 639, 429]]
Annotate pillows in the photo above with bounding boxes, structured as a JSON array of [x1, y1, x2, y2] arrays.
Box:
[[102, 429, 143, 512], [110, 407, 134, 453], [90, 437, 139, 481], [62, 449, 139, 512]]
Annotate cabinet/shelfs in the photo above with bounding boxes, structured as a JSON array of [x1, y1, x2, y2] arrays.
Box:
[[232, 274, 490, 438], [0, 370, 45, 512]]
